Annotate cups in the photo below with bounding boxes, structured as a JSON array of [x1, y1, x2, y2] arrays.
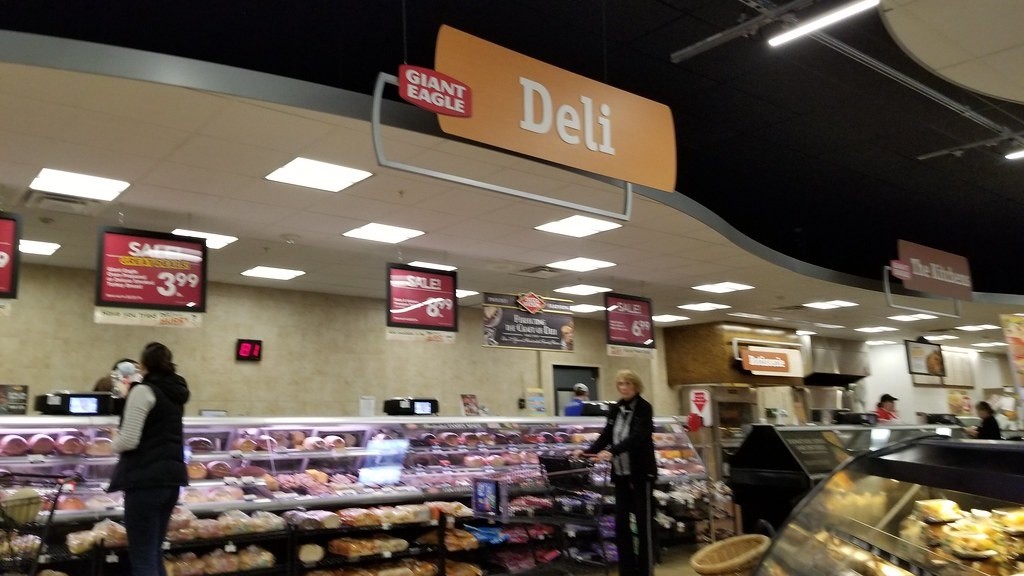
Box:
[[109, 372, 125, 398]]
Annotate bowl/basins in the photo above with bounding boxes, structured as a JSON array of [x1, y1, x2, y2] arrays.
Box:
[[957, 416, 983, 426]]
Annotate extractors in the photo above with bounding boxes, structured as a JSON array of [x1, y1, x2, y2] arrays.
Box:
[[799, 334, 871, 387]]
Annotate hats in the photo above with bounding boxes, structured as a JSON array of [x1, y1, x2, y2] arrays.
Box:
[[881, 394, 898, 403], [572, 382, 589, 392]]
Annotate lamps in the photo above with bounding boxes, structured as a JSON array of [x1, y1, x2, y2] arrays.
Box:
[[768, 0, 880, 47]]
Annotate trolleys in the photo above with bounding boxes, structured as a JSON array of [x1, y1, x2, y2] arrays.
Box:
[[0, 470, 87, 576], [473, 454, 611, 576]]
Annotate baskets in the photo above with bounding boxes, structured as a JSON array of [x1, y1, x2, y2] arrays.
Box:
[[689, 533, 772, 576]]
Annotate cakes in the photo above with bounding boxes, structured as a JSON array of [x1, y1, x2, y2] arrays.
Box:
[[911, 497, 1024, 554]]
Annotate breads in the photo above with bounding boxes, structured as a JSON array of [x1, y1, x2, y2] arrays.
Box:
[[0, 433, 585, 576]]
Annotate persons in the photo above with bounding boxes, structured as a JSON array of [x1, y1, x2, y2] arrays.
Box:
[[93, 342, 197, 576], [872, 395, 898, 421], [965, 393, 1024, 439], [562, 381, 589, 417], [572, 368, 660, 576]]
